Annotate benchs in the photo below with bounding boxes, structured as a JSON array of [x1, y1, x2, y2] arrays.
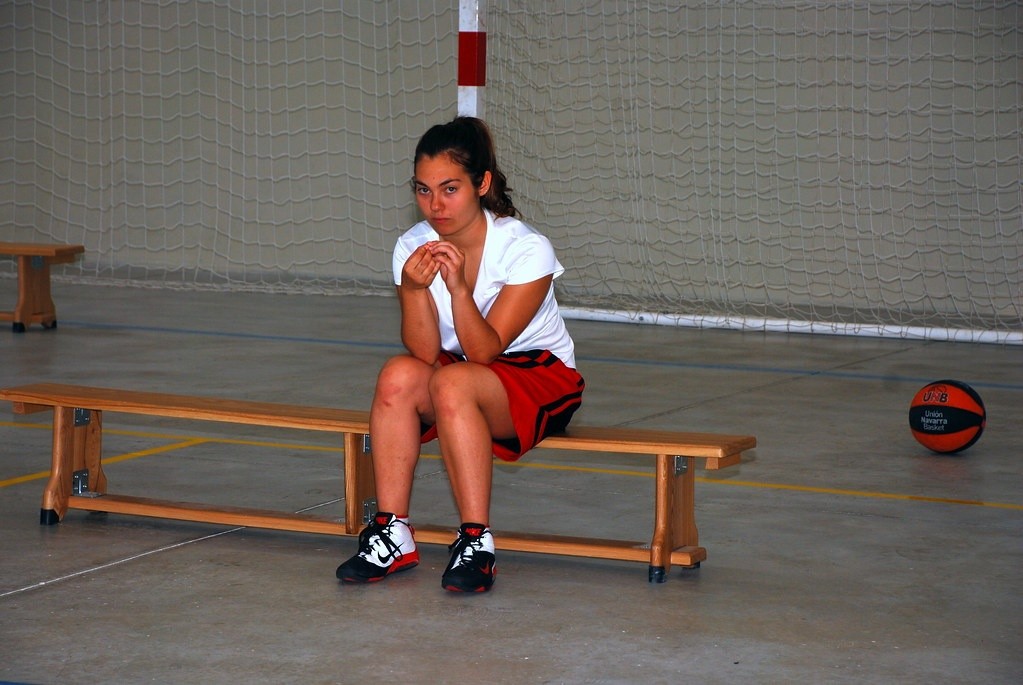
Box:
[[0, 382, 757, 584], [0, 241, 85, 334]]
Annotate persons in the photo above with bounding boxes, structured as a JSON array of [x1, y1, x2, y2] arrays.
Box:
[[335, 117, 585, 592]]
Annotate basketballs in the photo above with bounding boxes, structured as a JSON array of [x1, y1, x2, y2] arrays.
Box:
[[909, 379, 987, 454]]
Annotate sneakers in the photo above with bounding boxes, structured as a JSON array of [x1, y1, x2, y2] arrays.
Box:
[[337, 512, 420, 583], [442, 522, 497, 592]]
